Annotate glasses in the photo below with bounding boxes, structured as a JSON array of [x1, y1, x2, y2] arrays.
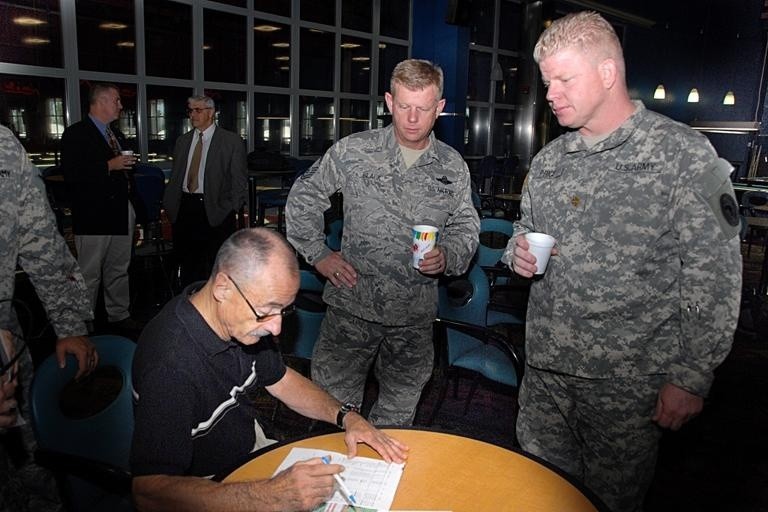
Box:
[[227, 274, 297, 324], [186, 107, 211, 113]]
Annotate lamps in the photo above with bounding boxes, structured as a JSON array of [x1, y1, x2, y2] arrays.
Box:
[[722, 31, 739, 105], [686, 27, 702, 102], [652, 23, 668, 100]]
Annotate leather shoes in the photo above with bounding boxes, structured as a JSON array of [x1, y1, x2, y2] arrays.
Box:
[[110, 317, 148, 335]]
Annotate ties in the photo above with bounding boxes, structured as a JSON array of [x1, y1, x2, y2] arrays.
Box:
[[187, 132, 202, 195], [106, 127, 119, 157]]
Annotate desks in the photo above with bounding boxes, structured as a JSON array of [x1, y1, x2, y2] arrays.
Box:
[[198, 423, 612, 511]]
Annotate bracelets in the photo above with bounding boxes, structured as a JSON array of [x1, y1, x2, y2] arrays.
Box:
[[336, 401, 359, 428]]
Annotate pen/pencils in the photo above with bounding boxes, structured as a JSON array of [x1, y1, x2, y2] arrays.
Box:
[[321, 456, 357, 504]]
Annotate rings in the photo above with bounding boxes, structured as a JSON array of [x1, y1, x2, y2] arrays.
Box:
[[90, 359, 96, 363], [333, 271, 341, 278]]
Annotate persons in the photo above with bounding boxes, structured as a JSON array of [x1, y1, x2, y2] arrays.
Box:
[[284, 58, 481, 426], [132, 226, 410, 511], [1, 125, 99, 510], [59, 83, 138, 335], [163, 95, 246, 292], [500, 12, 741, 512]]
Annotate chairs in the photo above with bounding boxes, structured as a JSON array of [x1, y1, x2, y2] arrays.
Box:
[[118, 153, 343, 364], [459, 140, 766, 258], [28, 331, 136, 512], [423, 264, 529, 430], [450, 216, 532, 401]]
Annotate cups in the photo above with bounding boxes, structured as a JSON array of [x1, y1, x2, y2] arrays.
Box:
[[524, 231, 556, 276], [120, 151, 133, 165], [411, 225, 440, 269]]
